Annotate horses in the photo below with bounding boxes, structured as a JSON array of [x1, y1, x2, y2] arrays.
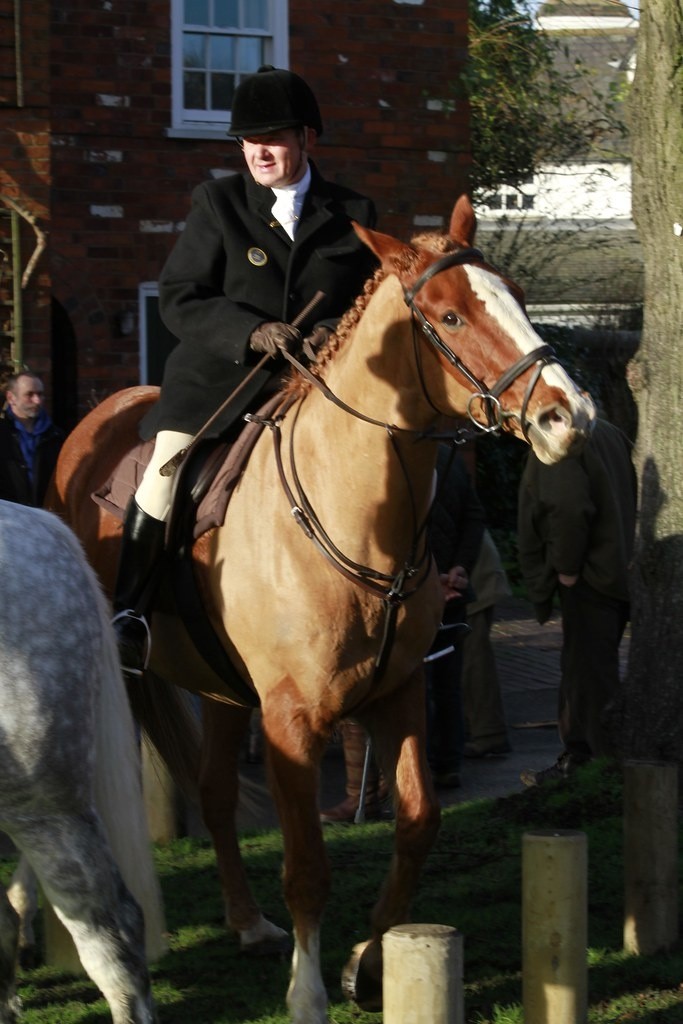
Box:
[[0, 193, 597, 1024]]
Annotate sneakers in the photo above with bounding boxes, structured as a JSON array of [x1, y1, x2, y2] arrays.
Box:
[[520, 748, 574, 787]]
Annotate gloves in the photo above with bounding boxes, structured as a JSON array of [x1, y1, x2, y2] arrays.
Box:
[[249, 322, 302, 360], [309, 327, 332, 352]]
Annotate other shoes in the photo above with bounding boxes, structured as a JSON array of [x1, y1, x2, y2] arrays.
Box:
[[430, 771, 461, 790], [465, 736, 512, 754]]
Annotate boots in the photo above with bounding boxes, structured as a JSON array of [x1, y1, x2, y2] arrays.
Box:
[[111, 495, 167, 668], [378, 770, 395, 819], [319, 723, 378, 822]]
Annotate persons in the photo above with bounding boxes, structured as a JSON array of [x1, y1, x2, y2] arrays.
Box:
[[110, 62, 382, 674], [319, 440, 516, 825], [0, 370, 61, 508], [518, 415, 637, 787]]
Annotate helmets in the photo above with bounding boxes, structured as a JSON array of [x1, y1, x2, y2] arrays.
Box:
[[226, 65, 323, 136]]
